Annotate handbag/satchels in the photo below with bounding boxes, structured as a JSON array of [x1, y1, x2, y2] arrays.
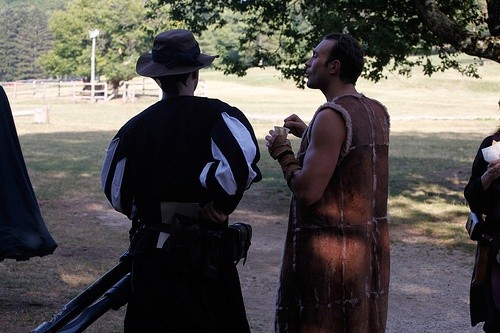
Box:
[[466, 211, 484, 241]]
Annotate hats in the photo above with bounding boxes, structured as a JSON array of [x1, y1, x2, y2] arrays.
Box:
[[137, 29, 219, 77]]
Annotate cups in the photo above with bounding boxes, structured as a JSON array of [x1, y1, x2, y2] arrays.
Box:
[[274, 126, 289, 136]]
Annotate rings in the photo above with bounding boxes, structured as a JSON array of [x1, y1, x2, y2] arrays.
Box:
[[490, 166, 495, 172]]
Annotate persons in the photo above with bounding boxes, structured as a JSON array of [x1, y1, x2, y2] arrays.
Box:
[[265, 34, 390, 333], [100, 30, 262, 331], [463, 99, 500, 333]]
